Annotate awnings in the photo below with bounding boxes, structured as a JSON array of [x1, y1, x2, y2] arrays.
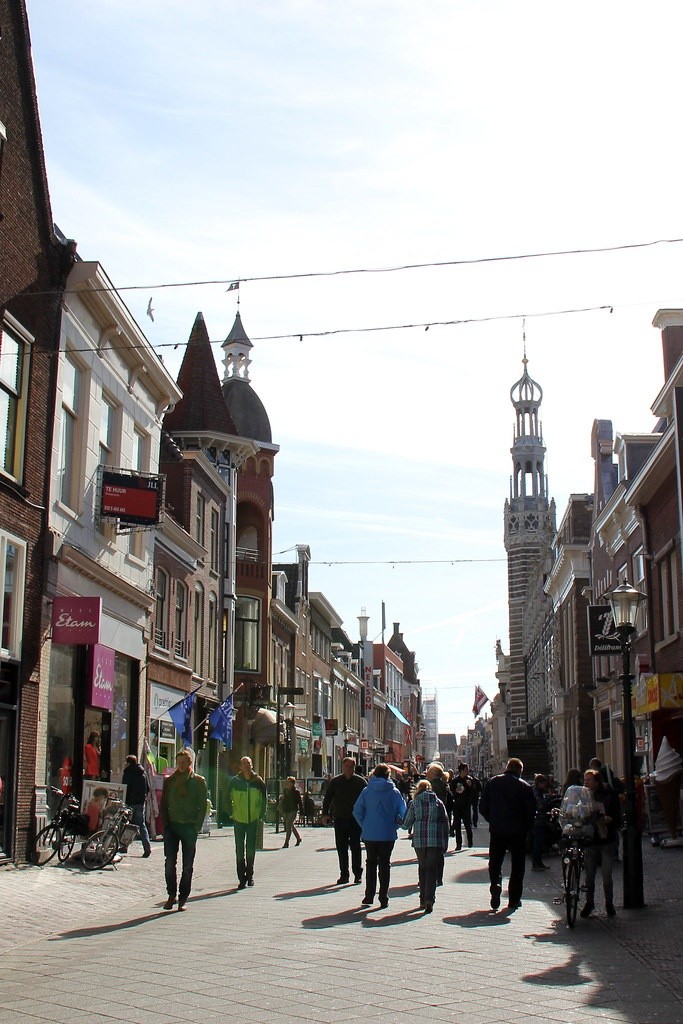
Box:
[[251, 707, 278, 747], [386, 702, 411, 727]]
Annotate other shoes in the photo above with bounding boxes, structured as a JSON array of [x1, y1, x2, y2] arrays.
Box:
[[379, 898, 389, 908], [143, 850, 151, 858], [247, 875, 254, 886], [295, 839, 301, 846], [467, 837, 473, 848], [282, 842, 289, 848], [117, 847, 127, 853], [354, 873, 362, 883], [178, 899, 187, 911], [337, 871, 349, 884], [425, 903, 433, 912], [420, 898, 425, 910], [490, 884, 502, 909], [164, 897, 176, 910], [455, 843, 462, 851], [238, 875, 247, 890], [362, 897, 373, 904], [508, 899, 522, 907]]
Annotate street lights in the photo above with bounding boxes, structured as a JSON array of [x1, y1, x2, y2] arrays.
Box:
[[280, 701, 297, 776], [603, 579, 646, 909]]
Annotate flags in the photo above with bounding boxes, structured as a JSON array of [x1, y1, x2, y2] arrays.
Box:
[[208, 693, 236, 749], [472, 685, 489, 719], [167, 688, 203, 745], [225, 282, 239, 291]]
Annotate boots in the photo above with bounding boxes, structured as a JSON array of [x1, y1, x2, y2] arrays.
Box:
[[580, 883, 595, 918], [604, 883, 615, 916], [531, 859, 551, 871]]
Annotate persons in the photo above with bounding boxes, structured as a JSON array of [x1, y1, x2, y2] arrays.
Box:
[[84, 731, 101, 781], [83, 788, 117, 830], [564, 769, 582, 788], [468, 769, 482, 828], [418, 761, 445, 886], [323, 757, 365, 884], [278, 777, 303, 848], [479, 758, 536, 909], [225, 756, 267, 891], [447, 769, 454, 779], [405, 779, 449, 912], [353, 765, 406, 908], [580, 769, 622, 918], [158, 750, 208, 911], [121, 754, 151, 858], [451, 764, 473, 851], [530, 774, 549, 869], [589, 758, 602, 770]]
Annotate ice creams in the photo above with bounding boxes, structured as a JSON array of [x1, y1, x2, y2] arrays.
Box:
[[654, 736, 682, 841]]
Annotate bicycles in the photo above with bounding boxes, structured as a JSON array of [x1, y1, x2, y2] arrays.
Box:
[[30, 786, 80, 866], [81, 796, 134, 869], [546, 809, 607, 927]]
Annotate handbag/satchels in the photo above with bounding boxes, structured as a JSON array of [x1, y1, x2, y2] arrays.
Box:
[[255, 820, 263, 850], [276, 798, 283, 816]]
